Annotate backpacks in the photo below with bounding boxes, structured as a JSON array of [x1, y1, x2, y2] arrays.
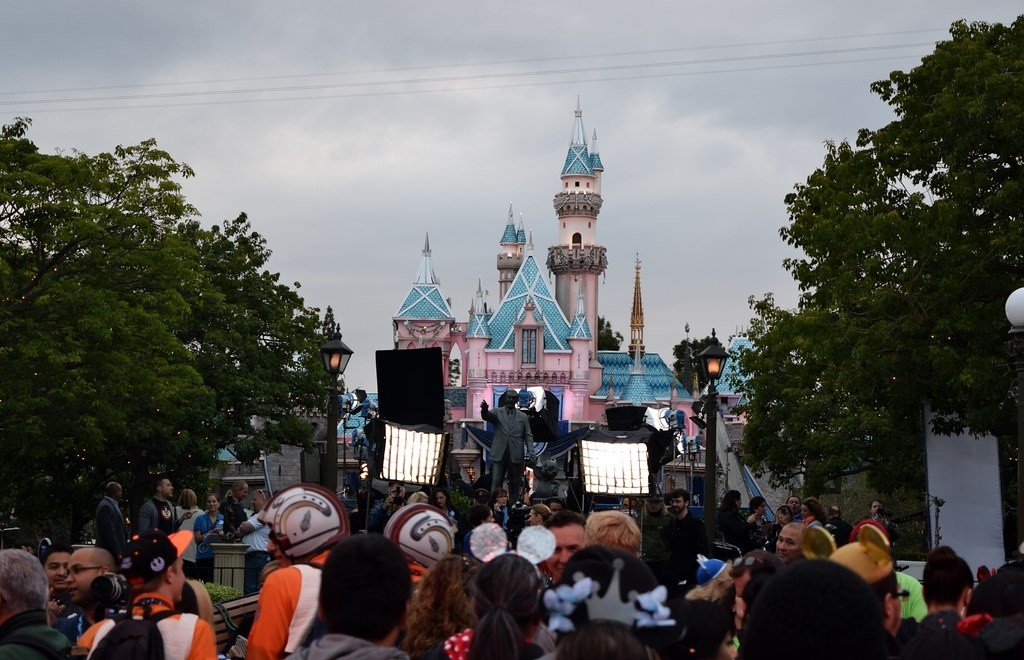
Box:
[[89, 602, 182, 660]]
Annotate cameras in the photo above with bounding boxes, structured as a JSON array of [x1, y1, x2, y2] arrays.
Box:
[[91, 576, 131, 606], [392, 487, 403, 506]]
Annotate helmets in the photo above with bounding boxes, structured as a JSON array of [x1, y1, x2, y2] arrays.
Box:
[[258, 483, 350, 568], [383, 503, 454, 568]]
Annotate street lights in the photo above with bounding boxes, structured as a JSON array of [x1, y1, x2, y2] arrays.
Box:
[[319, 322, 355, 495], [694, 328, 731, 558], [1005, 287, 1024, 560]]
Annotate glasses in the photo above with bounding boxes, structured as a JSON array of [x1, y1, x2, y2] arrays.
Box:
[[267, 529, 279, 547], [67, 564, 102, 576], [647, 500, 664, 506]]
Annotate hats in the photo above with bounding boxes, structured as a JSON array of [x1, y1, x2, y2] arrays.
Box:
[[660, 600, 728, 660], [645, 488, 666, 502], [737, 560, 903, 660], [802, 518, 893, 582], [695, 553, 727, 585], [557, 546, 687, 645], [473, 488, 490, 504], [117, 518, 193, 585]]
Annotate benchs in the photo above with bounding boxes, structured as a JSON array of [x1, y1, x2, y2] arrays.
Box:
[[213, 589, 259, 652]]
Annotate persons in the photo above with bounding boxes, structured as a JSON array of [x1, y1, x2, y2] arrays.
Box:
[[0, 478, 1024, 660], [480, 389, 537, 503], [526, 449, 560, 497]]
